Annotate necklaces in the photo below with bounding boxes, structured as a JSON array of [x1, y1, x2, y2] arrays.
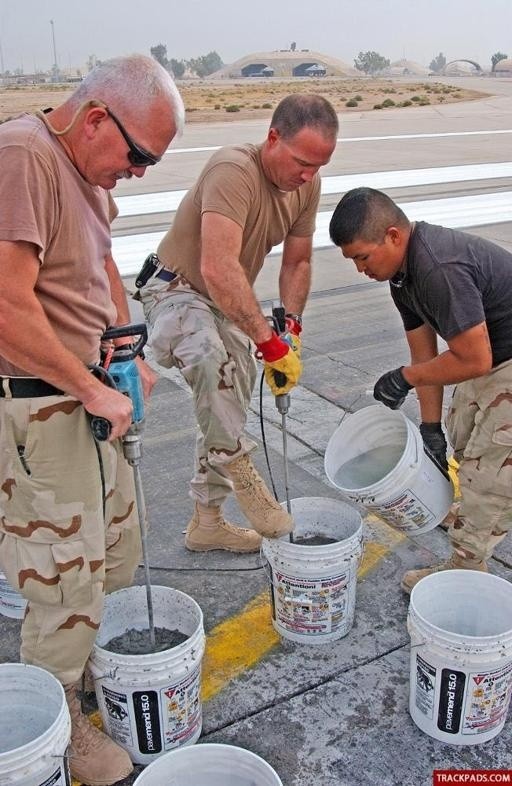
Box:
[[389, 271, 408, 288]]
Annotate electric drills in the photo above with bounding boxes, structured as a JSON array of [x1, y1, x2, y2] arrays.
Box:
[[263, 308, 294, 545], [89, 322, 156, 644]]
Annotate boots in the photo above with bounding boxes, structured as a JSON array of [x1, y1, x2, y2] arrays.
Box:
[[223, 454, 296, 538], [64, 685, 134, 786], [400, 551, 488, 595], [185, 500, 262, 554], [439, 500, 508, 561]]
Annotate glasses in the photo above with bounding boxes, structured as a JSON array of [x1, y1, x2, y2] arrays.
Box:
[[104, 106, 156, 167]]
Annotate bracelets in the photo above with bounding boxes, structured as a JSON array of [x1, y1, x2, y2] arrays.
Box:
[[286, 313, 303, 326], [115, 342, 146, 361]]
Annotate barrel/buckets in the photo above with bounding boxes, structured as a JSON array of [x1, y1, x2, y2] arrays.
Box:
[[0, 662, 78, 786], [323, 390, 456, 538], [260, 495, 364, 645], [86, 584, 207, 766], [132, 743, 283, 786], [405, 568, 512, 747]]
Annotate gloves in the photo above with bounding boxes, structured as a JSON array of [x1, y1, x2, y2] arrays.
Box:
[[420, 422, 449, 470], [374, 365, 415, 410], [284, 316, 302, 357], [254, 330, 301, 395]]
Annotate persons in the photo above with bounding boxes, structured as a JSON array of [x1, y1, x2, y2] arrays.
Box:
[[0, 54, 185, 786], [131, 92, 339, 554], [330, 187, 512, 594]]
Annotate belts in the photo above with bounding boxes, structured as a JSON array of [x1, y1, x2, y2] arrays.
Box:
[[156, 268, 176, 281], [0, 376, 65, 397]]
